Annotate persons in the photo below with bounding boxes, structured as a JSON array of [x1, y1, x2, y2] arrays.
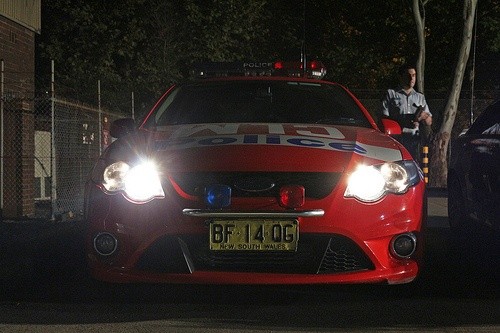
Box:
[[379, 65, 432, 157]]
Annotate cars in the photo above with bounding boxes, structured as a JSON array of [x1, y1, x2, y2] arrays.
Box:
[[447, 101, 500, 261], [75, 72, 428, 292]]
